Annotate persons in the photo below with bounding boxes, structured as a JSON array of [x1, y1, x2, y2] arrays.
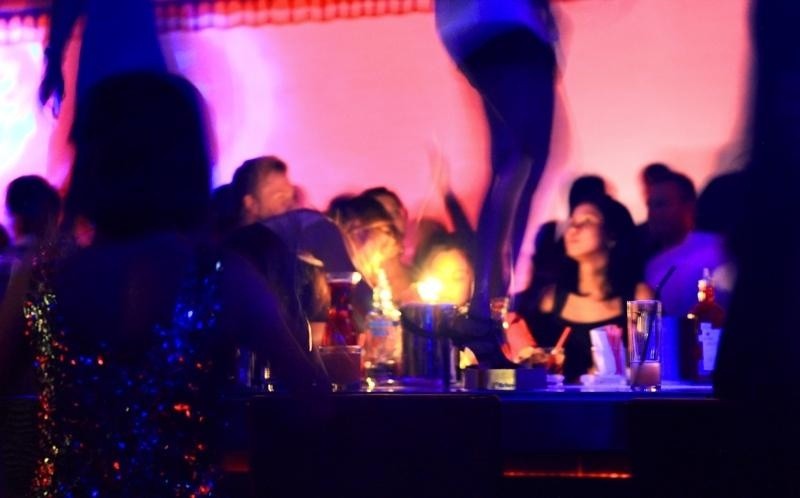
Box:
[[525, 175, 607, 285], [642, 171, 767, 375], [436, 1, 561, 324], [37, 1, 166, 244], [630, 162, 698, 275], [1, 64, 476, 497], [500, 193, 655, 370]]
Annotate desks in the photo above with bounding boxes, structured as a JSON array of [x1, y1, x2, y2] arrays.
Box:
[[241, 381, 717, 498]]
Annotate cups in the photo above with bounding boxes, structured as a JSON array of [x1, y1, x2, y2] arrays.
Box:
[[545, 345, 566, 383], [626, 298, 664, 392], [317, 344, 362, 392], [483, 368, 518, 390], [489, 295, 513, 331]]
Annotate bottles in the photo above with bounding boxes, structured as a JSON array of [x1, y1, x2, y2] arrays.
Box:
[[677, 282, 730, 385], [320, 271, 363, 345]]
